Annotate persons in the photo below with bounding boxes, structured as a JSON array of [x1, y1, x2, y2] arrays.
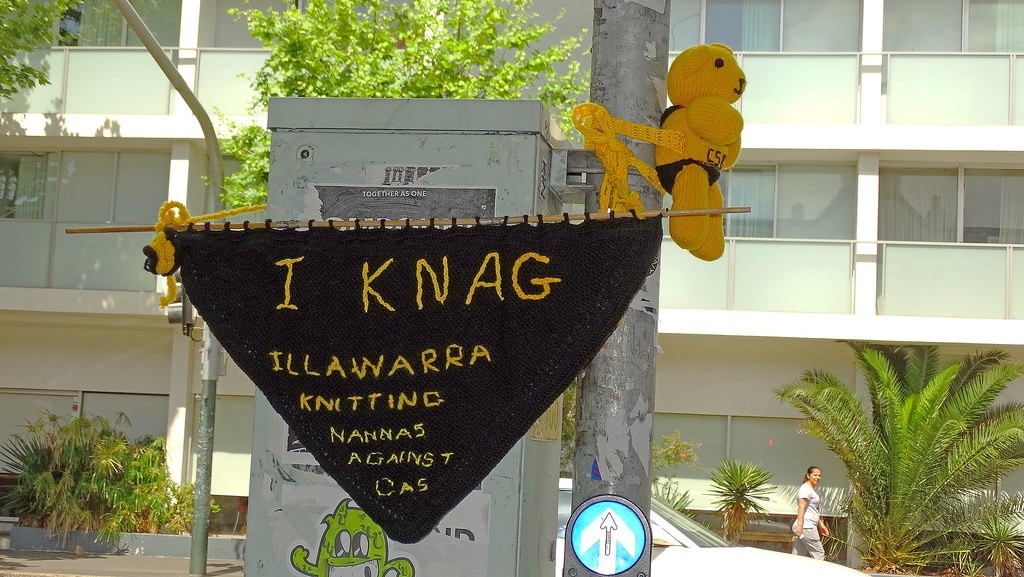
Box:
[[790, 466, 829, 560]]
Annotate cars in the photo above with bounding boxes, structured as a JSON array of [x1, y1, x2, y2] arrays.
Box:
[[555, 474, 873, 577]]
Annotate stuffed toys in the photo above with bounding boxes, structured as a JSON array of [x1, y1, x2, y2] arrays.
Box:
[[655, 43, 747, 261]]
